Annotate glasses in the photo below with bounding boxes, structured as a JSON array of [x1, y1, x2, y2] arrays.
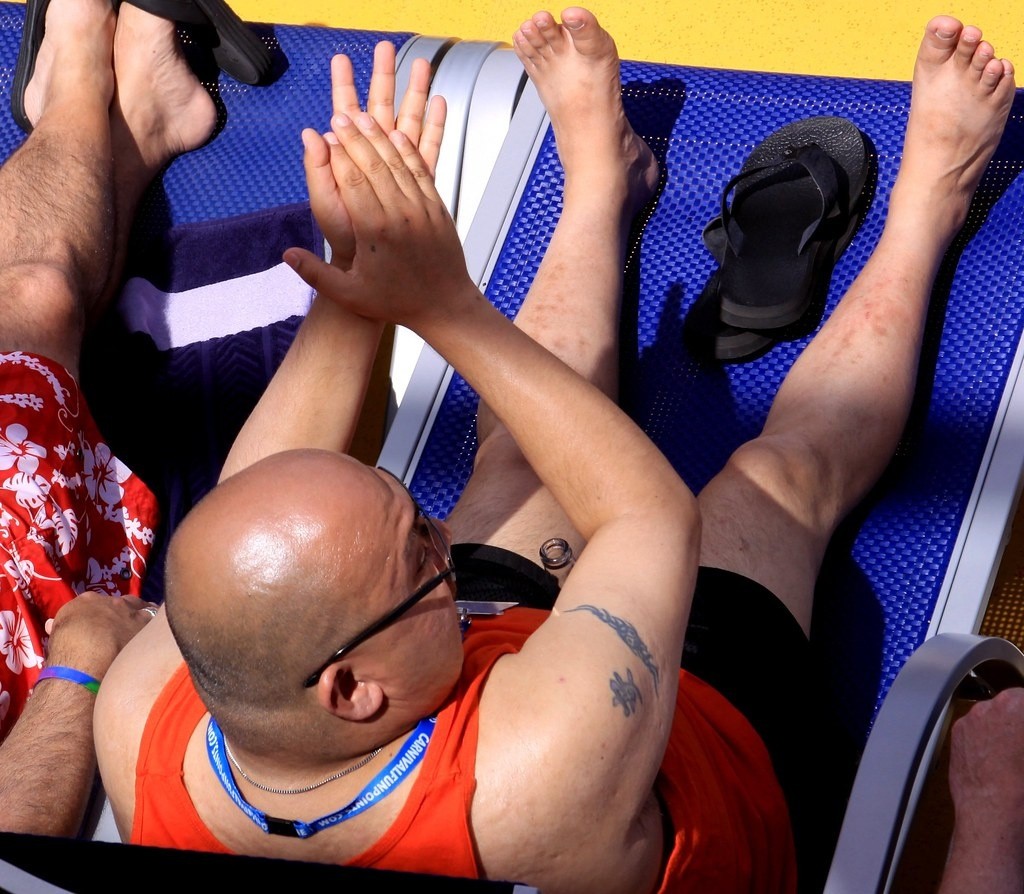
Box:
[[301, 466, 456, 688]]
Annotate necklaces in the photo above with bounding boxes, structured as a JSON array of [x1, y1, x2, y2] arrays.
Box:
[[222, 731, 384, 793]]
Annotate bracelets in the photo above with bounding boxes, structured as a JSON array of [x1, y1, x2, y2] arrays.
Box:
[[33, 666, 101, 696]]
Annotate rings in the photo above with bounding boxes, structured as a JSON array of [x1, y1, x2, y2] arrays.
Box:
[[143, 607, 161, 618]]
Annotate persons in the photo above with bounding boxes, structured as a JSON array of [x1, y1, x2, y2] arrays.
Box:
[[93, 6, 1016, 894], [0, 0, 278, 840], [942, 687, 1024, 894]]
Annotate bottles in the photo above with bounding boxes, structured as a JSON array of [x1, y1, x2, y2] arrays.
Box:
[[539, 538, 576, 590]]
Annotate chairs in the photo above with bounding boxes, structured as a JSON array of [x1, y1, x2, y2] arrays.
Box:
[[0, 3, 1024, 893]]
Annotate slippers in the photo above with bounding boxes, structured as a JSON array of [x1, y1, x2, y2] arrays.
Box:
[[12, 0, 53, 134], [684, 116, 870, 361], [125, 0, 272, 86]]
